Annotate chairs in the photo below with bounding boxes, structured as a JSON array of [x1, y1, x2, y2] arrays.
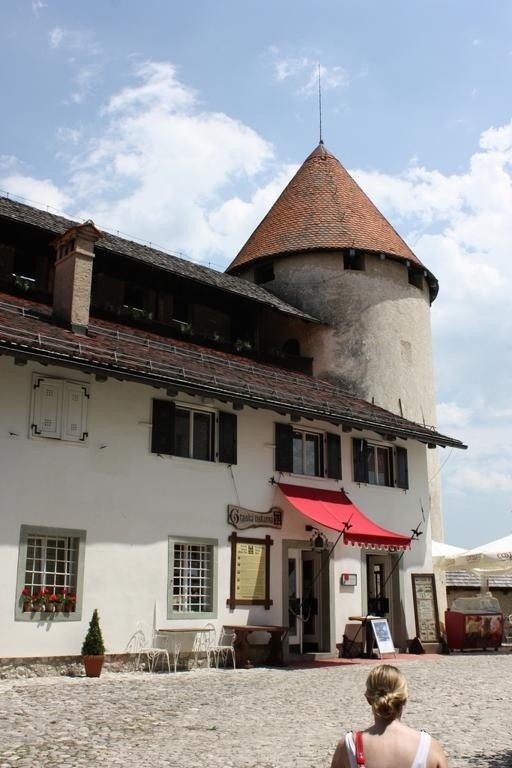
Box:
[[131, 621, 237, 674]]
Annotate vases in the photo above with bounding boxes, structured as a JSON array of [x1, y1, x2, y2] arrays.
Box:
[[23, 602, 74, 613]]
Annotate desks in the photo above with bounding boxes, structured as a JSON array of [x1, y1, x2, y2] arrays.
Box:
[[223, 623, 291, 670]]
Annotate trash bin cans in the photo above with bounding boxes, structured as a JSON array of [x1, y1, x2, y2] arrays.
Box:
[[445, 608, 503, 652]]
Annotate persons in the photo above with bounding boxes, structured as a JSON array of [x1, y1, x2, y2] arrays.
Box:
[[328, 663, 451, 768]]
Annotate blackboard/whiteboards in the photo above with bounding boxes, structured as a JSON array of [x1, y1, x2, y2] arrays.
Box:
[[370, 618, 396, 654]]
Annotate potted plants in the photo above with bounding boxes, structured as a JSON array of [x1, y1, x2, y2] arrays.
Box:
[[80, 607, 106, 678]]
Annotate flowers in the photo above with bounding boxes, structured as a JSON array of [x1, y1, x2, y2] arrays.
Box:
[[22, 587, 76, 603]]
